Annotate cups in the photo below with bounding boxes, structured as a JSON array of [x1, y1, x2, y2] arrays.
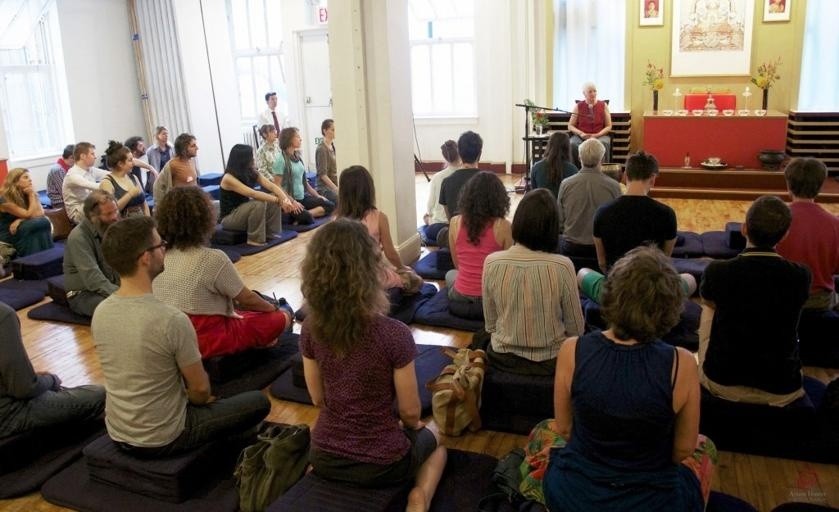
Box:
[[722, 110, 734, 116], [754, 110, 766, 117], [708, 158, 721, 165], [707, 110, 719, 117], [678, 110, 688, 116], [738, 110, 751, 116], [692, 110, 704, 117], [663, 110, 673, 117]]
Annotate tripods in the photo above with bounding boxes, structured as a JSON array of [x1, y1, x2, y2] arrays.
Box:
[[507, 104, 587, 194]]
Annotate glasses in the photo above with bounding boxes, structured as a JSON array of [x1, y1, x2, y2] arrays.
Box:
[[136, 240, 169, 259], [96, 207, 120, 216]]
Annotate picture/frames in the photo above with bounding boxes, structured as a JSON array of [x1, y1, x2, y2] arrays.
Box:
[[671, 0, 754, 78], [640, 1, 663, 27], [762, 0, 792, 22]]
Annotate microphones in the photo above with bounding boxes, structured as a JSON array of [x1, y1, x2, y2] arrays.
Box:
[[588, 104, 594, 123]]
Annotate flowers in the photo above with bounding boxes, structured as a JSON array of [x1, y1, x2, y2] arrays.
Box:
[[640, 59, 665, 89], [752, 56, 782, 89], [523, 99, 552, 128]]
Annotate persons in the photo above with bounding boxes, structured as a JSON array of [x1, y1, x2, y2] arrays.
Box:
[[299, 217, 448, 512], [437, 131, 483, 249], [520, 247, 718, 512], [556, 137, 625, 263], [775, 158, 839, 309], [331, 166, 422, 308], [91, 216, 271, 460], [645, 1, 659, 18], [567, 83, 611, 168], [272, 128, 335, 225], [482, 187, 585, 386], [124, 137, 159, 197], [152, 185, 289, 360], [63, 143, 112, 227], [256, 92, 289, 137], [576, 150, 697, 305], [0, 168, 53, 257], [418, 139, 463, 247], [219, 144, 294, 245], [315, 119, 339, 208], [770, 0, 786, 13], [445, 172, 513, 319], [1, 301, 106, 451], [146, 126, 175, 174], [99, 141, 151, 217], [253, 124, 281, 194], [152, 134, 198, 202], [530, 132, 579, 200], [46, 145, 75, 209]]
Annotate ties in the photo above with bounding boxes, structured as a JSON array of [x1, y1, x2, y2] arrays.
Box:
[[272, 111, 282, 137]]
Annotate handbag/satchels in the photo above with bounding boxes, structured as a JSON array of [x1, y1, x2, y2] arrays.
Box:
[[428, 346, 487, 435], [477, 448, 533, 512]]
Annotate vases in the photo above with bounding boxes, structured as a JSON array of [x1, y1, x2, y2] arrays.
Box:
[[535, 128, 548, 135], [762, 88, 768, 113], [653, 89, 659, 114]]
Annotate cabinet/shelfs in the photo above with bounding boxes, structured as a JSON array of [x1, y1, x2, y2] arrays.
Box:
[[643, 110, 789, 168]]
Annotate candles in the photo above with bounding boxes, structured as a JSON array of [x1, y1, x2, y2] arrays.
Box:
[[675, 88, 678, 92], [745, 86, 750, 92]]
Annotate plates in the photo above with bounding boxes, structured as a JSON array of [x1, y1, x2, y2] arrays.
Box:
[[698, 159, 728, 169]]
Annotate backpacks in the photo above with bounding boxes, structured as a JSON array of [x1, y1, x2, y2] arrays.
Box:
[[694, 196, 812, 407], [63, 189, 121, 318]]
